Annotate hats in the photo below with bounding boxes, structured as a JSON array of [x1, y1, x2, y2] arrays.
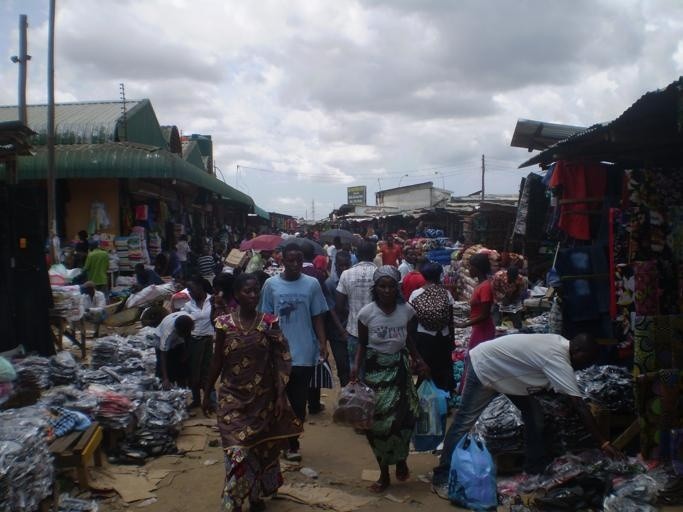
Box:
[[81, 281, 95, 289]]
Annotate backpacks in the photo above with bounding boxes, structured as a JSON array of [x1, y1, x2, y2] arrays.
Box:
[[412, 284, 449, 331]]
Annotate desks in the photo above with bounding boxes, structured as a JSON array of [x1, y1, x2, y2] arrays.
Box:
[[50, 311, 86, 359]]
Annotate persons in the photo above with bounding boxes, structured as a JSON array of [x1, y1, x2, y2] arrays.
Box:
[[198, 273, 306, 511], [429, 332, 630, 499], [80, 237, 110, 306], [69, 230, 89, 268], [134, 263, 166, 288], [72, 280, 108, 337], [144, 217, 564, 423], [347, 265, 432, 493]]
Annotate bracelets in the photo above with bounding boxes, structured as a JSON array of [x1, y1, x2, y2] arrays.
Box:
[[73, 250, 77, 255], [600, 440, 611, 449]]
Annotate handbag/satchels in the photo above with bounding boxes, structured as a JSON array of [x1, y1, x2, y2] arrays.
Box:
[[225, 248, 247, 267]]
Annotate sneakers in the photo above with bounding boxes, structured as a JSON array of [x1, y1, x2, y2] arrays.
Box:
[[312, 402, 324, 415], [283, 448, 302, 461], [431, 484, 449, 499]]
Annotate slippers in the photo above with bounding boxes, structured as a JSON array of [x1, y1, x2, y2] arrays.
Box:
[[367, 478, 390, 493], [396, 462, 409, 481]]
